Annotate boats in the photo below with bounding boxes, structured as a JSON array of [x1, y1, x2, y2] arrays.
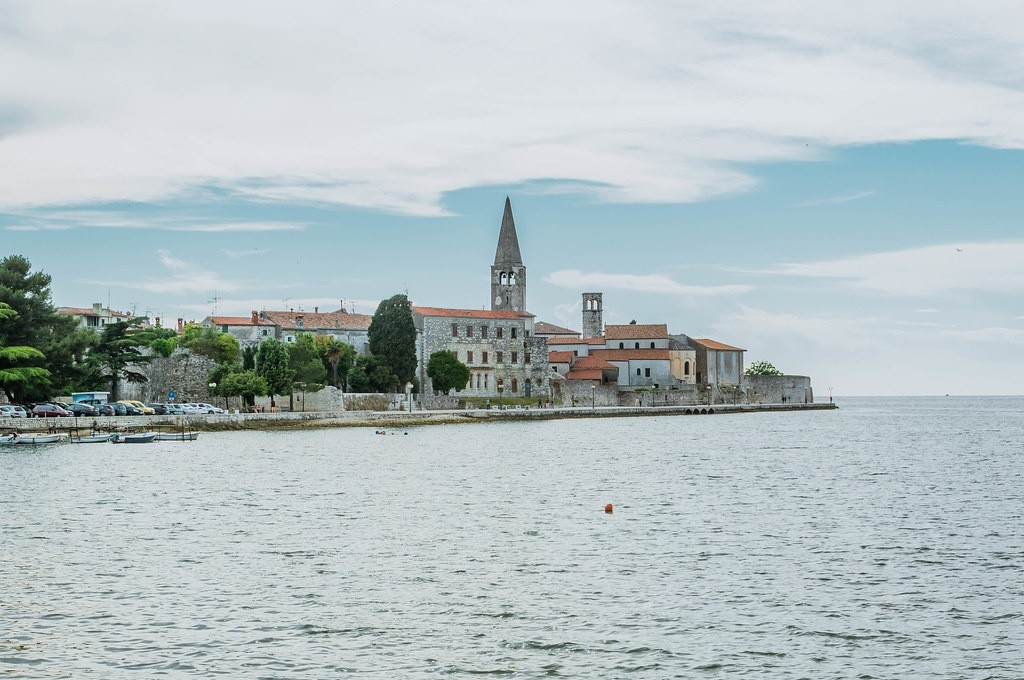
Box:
[[109, 424, 159, 443], [150, 409, 201, 440], [0, 421, 60, 445], [67, 417, 112, 443]]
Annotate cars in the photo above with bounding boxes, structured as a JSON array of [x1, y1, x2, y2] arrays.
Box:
[[53, 400, 128, 417], [32, 403, 75, 418], [117, 400, 155, 415], [116, 401, 145, 416], [148, 401, 224, 415], [0, 403, 36, 421]]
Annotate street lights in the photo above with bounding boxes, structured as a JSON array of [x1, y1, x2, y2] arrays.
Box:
[[706, 386, 712, 406], [733, 386, 737, 406], [406, 381, 414, 413], [652, 385, 656, 407], [208, 382, 216, 406], [804, 387, 808, 403], [591, 385, 596, 409], [497, 384, 504, 410]]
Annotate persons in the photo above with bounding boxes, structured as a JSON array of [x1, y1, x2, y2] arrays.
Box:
[[486, 398, 490, 410], [239, 403, 257, 413], [537, 397, 554, 409], [781, 394, 791, 405], [271, 400, 275, 413], [8, 423, 57, 440], [634, 396, 642, 407]]
